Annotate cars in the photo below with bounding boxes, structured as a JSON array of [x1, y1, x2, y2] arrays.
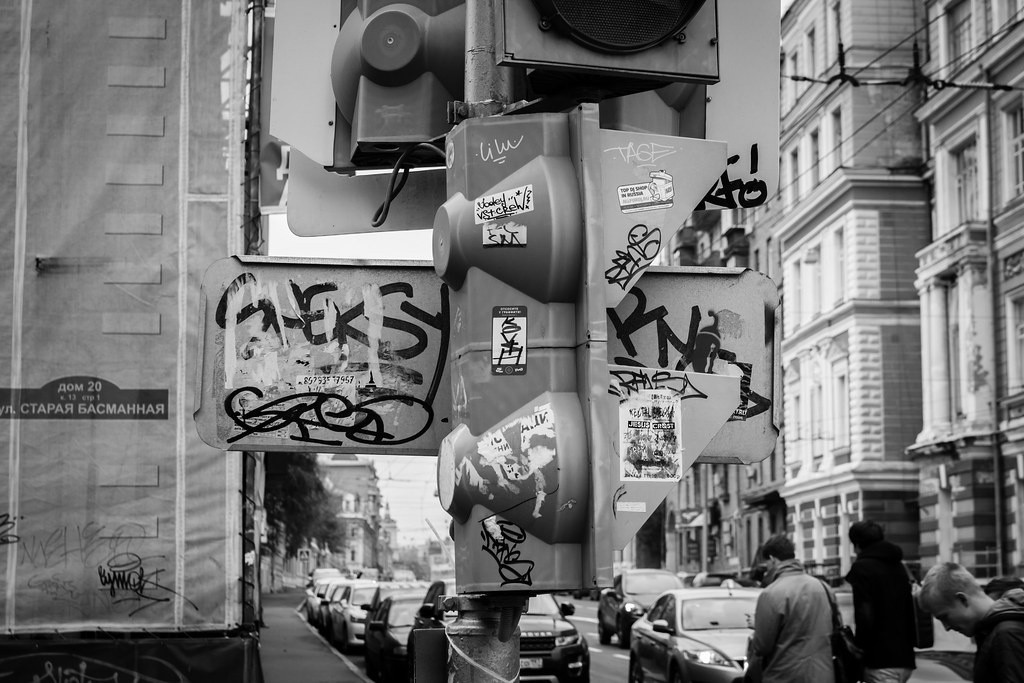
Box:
[[368, 592, 432, 683], [629, 584, 768, 683], [360, 585, 429, 666], [406, 579, 591, 683], [596, 568, 689, 649], [333, 582, 381, 655], [831, 592, 972, 682], [305, 567, 416, 638]]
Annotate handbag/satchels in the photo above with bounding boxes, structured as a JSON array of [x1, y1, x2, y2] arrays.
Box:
[[901, 560, 935, 649], [820, 580, 863, 683]]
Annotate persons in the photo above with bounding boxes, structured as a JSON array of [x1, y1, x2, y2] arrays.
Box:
[[744, 536, 842, 683], [844, 522, 917, 683], [918, 562, 1024, 683]]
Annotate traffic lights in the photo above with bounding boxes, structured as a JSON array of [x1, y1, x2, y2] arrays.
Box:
[[575, 102, 745, 584]]
